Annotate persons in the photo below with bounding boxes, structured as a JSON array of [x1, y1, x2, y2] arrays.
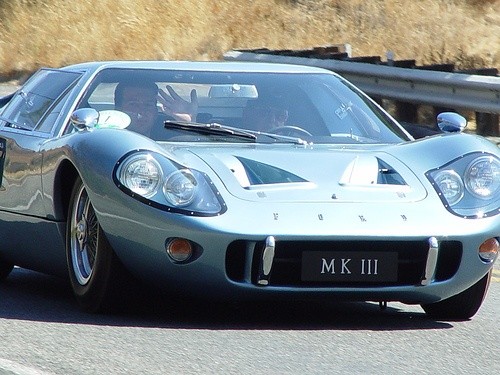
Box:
[[154, 84, 312, 145], [114, 76, 159, 139]]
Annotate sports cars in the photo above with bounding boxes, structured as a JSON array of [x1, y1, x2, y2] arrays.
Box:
[[0, 61, 500, 321]]
